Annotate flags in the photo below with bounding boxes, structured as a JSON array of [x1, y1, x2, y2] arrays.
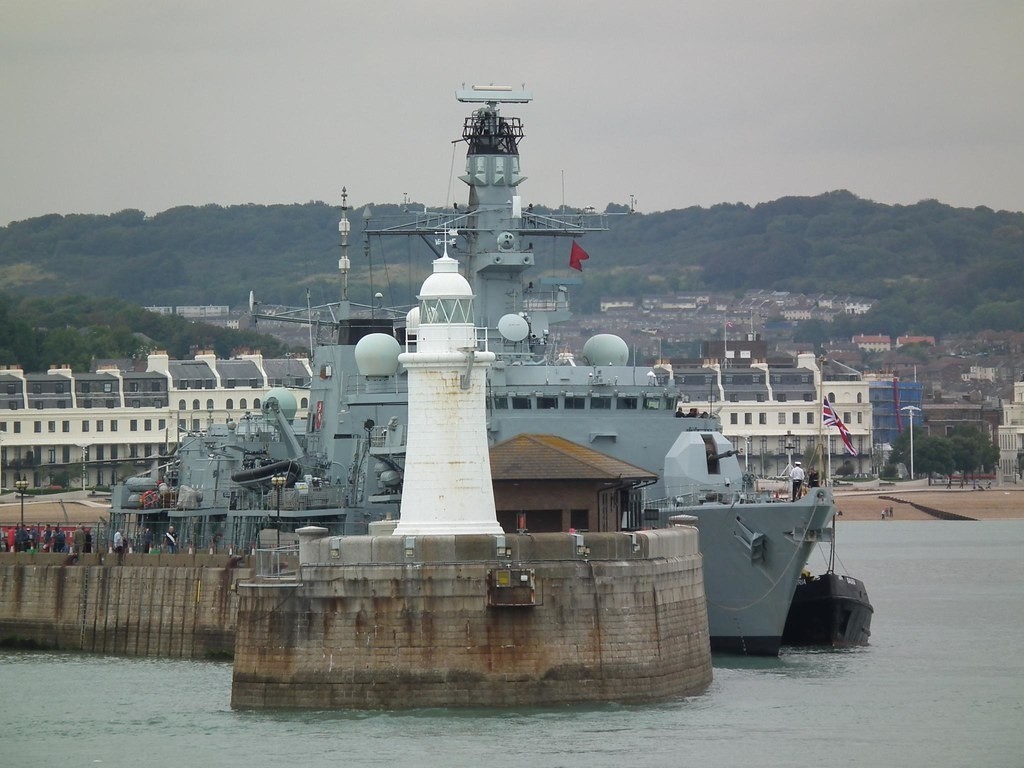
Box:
[[725, 320, 733, 328], [569, 240, 589, 272], [823, 395, 857, 456]]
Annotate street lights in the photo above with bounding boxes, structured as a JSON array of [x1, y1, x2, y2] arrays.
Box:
[[16, 480, 29, 531], [900, 405, 922, 479]]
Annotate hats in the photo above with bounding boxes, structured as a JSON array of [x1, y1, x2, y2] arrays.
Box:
[[795, 462, 801, 464]]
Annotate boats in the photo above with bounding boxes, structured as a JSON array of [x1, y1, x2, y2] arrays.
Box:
[[780, 513, 874, 650], [102, 79, 843, 659]]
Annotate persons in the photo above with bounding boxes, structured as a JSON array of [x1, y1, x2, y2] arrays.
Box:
[[885, 504, 889, 517], [676, 406, 699, 418], [144, 528, 152, 553], [986, 479, 991, 489], [890, 505, 893, 517], [8, 523, 91, 553], [959, 478, 964, 488], [790, 462, 804, 502], [164, 526, 178, 554], [946, 477, 951, 489], [881, 508, 885, 519], [808, 467, 823, 497], [978, 479, 984, 489], [114, 529, 124, 565]]
